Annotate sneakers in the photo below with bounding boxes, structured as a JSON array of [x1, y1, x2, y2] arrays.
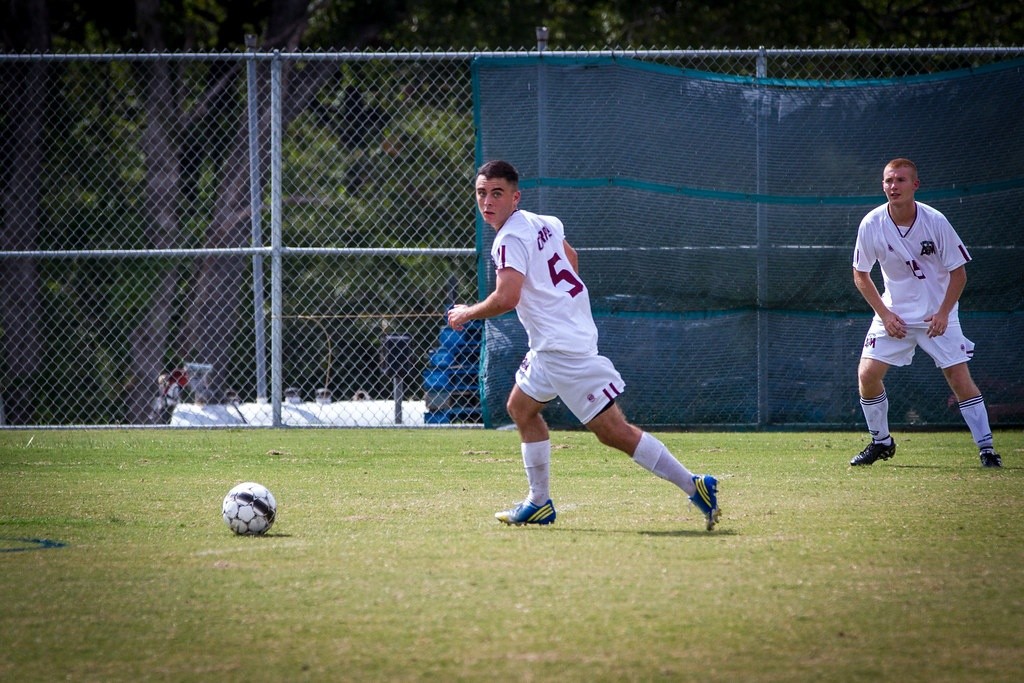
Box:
[[492, 499, 557, 526], [977, 450, 1004, 471], [687, 476, 723, 531], [851, 437, 897, 466]]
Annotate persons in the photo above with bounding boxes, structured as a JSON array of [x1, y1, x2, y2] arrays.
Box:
[[850, 158, 1002, 469], [447, 160, 722, 530]]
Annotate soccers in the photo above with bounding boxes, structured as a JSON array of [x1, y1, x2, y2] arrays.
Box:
[[222, 481, 277, 535]]
